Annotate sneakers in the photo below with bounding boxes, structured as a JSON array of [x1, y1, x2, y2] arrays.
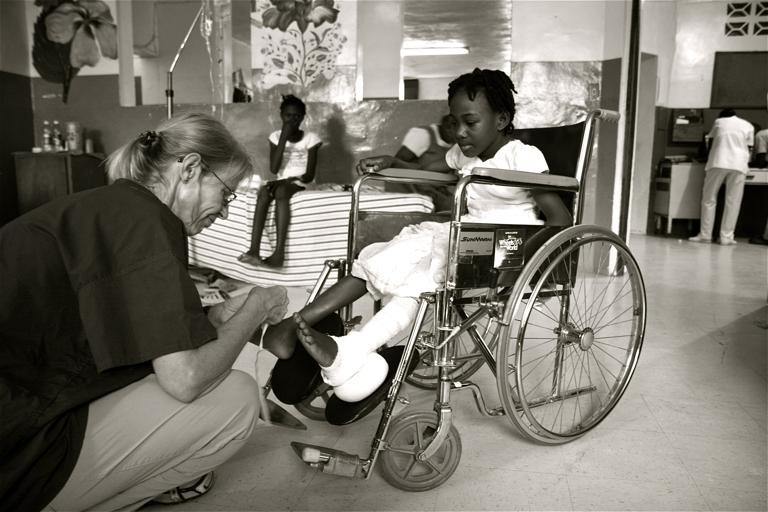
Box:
[[153, 471, 215, 503]]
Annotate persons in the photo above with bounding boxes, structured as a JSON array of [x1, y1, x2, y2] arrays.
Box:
[[243, 68, 572, 388], [237, 94, 321, 272], [748, 126, 768, 246], [0, 113, 289, 511], [689, 106, 756, 245], [387, 113, 462, 218]]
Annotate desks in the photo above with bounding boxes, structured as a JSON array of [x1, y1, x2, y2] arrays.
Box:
[[723, 167, 768, 186]]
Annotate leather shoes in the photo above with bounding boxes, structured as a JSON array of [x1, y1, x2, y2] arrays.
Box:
[[689, 235, 711, 242]]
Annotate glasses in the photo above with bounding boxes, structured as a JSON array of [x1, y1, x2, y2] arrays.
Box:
[[177, 156, 237, 203]]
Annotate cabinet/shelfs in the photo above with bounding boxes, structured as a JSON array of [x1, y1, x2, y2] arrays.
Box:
[[11, 150, 107, 216], [653, 162, 706, 235]]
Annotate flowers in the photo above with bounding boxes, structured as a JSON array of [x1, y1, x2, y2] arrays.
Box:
[[36, 0, 117, 103], [258, 0, 348, 100]]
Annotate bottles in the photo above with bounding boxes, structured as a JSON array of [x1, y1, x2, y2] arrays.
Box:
[[41, 119, 53, 151], [53, 119, 63, 153]]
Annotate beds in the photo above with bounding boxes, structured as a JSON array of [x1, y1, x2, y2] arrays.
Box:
[[185, 175, 436, 289]]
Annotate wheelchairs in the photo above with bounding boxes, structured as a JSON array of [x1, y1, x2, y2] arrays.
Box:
[[250, 107, 648, 489]]
